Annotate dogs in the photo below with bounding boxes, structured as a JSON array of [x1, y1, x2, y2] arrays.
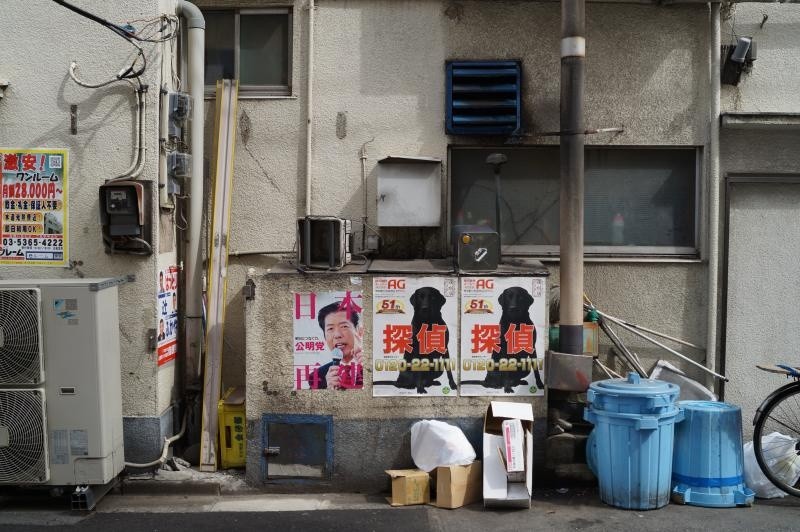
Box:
[[372, 286, 457, 395], [460, 286, 544, 394]]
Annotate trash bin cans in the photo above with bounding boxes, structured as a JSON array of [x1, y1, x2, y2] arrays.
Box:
[[583, 371, 685, 508], [671, 399, 756, 507]]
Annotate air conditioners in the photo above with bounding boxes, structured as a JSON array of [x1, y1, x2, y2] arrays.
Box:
[[0, 279, 124, 485]]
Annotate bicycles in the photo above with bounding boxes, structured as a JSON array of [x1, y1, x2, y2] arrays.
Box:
[[752, 364, 800, 500]]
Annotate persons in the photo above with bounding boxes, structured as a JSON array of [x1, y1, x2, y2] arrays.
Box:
[[172, 293, 177, 313], [158, 319, 165, 340], [159, 272, 164, 293], [308, 301, 363, 389]]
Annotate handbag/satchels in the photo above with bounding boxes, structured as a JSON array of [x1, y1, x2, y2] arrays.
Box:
[[743, 432, 800, 498]]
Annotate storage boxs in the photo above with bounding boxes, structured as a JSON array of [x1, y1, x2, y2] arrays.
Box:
[[384, 402, 538, 511], [218, 389, 247, 467]]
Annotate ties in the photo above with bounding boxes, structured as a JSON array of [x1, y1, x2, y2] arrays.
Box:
[[345, 364, 351, 384]]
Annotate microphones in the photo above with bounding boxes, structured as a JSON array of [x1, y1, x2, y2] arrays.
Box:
[[331, 348, 344, 390]]
